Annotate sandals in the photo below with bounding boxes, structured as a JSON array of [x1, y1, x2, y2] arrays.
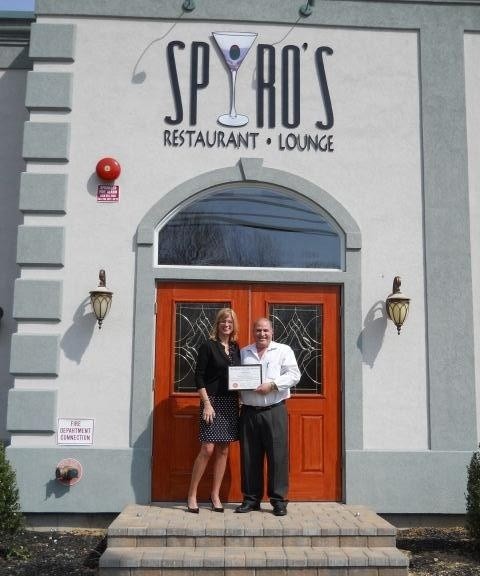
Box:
[[187, 496, 200, 514]]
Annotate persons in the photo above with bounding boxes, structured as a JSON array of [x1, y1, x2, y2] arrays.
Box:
[[186, 307, 241, 515], [236, 317, 302, 516]]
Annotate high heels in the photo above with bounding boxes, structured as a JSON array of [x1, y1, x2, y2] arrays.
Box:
[[209, 494, 225, 513]]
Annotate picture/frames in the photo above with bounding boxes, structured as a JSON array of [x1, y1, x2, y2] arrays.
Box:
[[226, 364, 262, 391]]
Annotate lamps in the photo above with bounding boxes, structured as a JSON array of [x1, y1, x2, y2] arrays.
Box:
[[89, 270, 113, 329], [386, 277, 410, 335]]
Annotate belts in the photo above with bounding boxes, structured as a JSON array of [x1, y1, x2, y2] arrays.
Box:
[[242, 399, 286, 411]]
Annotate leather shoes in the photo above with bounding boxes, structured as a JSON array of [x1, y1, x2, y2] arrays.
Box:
[[273, 504, 288, 516], [235, 499, 261, 513]]
